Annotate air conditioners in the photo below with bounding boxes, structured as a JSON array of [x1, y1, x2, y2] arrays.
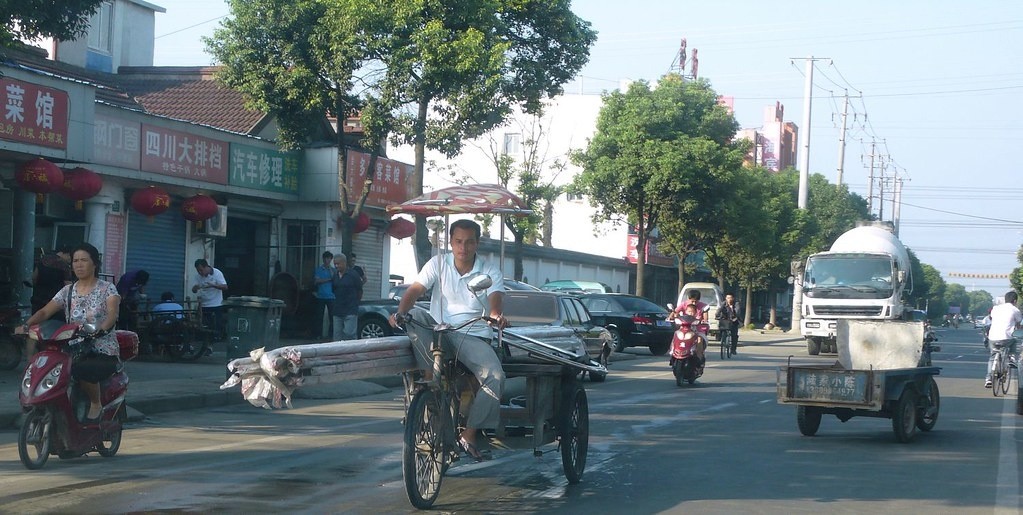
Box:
[[194, 205, 228, 238]]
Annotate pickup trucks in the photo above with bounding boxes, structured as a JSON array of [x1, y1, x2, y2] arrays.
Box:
[[357, 285, 431, 339]]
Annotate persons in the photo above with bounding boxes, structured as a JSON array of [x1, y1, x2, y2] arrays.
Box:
[[23, 244, 72, 360], [148, 290, 184, 323], [941, 308, 1023, 336], [330, 253, 363, 339], [389, 220, 509, 460], [313, 250, 335, 337], [349, 254, 367, 284], [116, 270, 149, 329], [15, 243, 120, 422], [715, 293, 741, 355], [674, 304, 702, 354], [666, 291, 709, 363], [192, 258, 227, 339], [984, 292, 1022, 387]]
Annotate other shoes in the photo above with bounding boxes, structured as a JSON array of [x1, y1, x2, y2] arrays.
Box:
[[1009, 361, 1018, 368], [82, 403, 104, 424], [984, 381, 992, 388]]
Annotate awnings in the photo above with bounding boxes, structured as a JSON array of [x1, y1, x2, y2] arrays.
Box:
[[385, 183, 534, 363]]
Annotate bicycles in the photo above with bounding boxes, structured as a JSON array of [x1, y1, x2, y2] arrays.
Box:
[[717, 315, 737, 360], [991, 343, 1013, 396]]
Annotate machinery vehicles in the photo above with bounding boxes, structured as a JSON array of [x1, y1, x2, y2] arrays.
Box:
[[787, 226, 913, 354]]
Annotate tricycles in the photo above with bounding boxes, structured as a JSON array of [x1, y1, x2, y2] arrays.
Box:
[[395, 274, 591, 509], [776, 319, 942, 444]]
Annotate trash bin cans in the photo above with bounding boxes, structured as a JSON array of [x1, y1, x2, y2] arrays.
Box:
[[227, 295, 285, 364]]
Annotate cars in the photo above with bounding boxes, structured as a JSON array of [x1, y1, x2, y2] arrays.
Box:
[[499, 289, 613, 365], [576, 293, 676, 357], [974, 315, 984, 326]]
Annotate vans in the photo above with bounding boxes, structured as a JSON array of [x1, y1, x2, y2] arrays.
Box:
[[677, 282, 724, 334], [542, 280, 612, 296]]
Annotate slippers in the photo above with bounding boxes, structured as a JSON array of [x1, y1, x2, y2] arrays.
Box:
[[456, 435, 483, 463]]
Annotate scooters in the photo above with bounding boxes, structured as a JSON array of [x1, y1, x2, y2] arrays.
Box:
[[943, 313, 972, 328], [665, 302, 711, 386], [19, 317, 139, 471]]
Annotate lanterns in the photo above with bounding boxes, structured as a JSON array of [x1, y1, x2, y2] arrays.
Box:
[[16, 156, 64, 205], [181, 194, 218, 229], [386, 215, 416, 243], [60, 166, 102, 212], [347, 210, 370, 238], [130, 187, 170, 222]]
